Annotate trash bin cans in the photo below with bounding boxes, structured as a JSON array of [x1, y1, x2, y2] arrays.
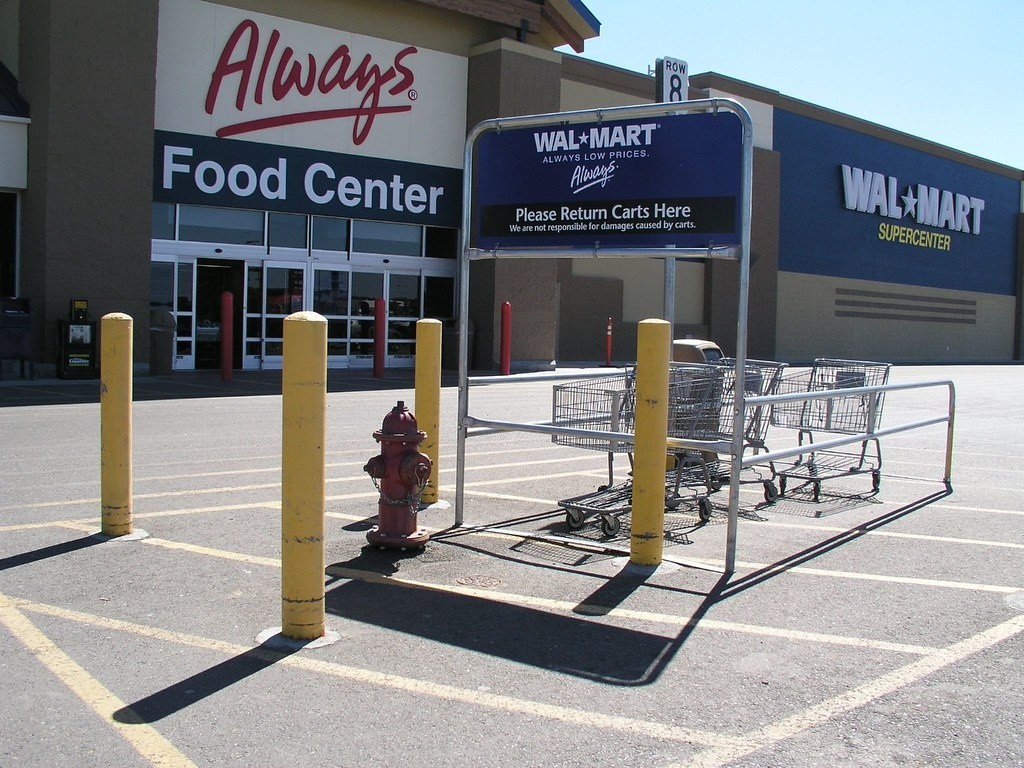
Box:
[[443, 317, 476, 371]]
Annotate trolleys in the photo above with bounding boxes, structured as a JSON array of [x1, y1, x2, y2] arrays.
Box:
[[551, 352, 894, 537]]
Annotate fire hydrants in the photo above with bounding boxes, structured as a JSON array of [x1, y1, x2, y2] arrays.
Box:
[[364, 400, 433, 549]]
